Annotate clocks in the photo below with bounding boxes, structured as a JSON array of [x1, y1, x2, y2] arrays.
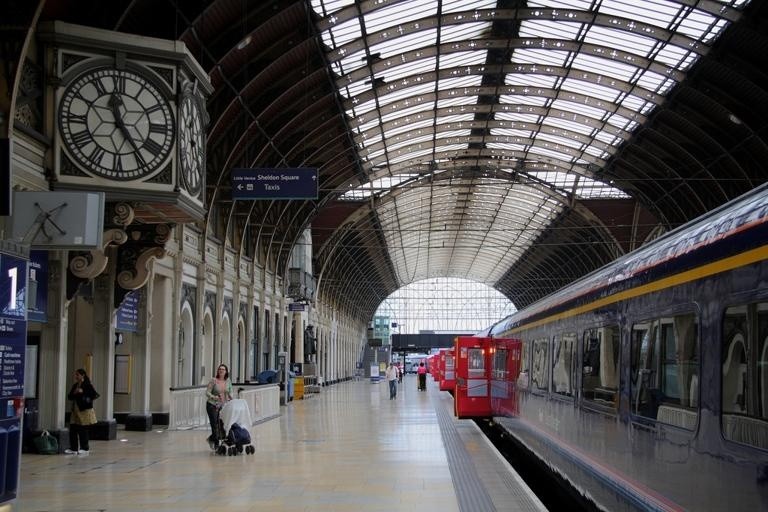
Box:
[[58, 65, 177, 181], [176, 92, 210, 196]]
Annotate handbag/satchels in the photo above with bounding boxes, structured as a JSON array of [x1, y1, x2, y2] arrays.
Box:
[[34, 431, 58, 455]]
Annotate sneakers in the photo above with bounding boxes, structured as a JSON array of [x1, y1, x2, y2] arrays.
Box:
[[78, 449, 90, 455], [206, 436, 215, 451], [64, 449, 78, 454]]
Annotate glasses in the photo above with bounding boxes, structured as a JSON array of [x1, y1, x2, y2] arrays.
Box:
[[218, 367, 225, 371]]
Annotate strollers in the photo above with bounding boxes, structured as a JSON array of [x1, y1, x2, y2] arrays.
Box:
[[217, 399, 255, 456]]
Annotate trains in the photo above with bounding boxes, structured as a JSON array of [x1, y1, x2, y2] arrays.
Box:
[[473, 181, 768, 512]]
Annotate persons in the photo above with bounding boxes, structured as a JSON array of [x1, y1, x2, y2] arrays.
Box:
[[385, 362, 399, 400], [204, 363, 234, 452], [397, 362, 402, 383], [63, 368, 94, 454], [305, 323, 318, 363], [417, 362, 428, 392], [393, 363, 398, 377]]
